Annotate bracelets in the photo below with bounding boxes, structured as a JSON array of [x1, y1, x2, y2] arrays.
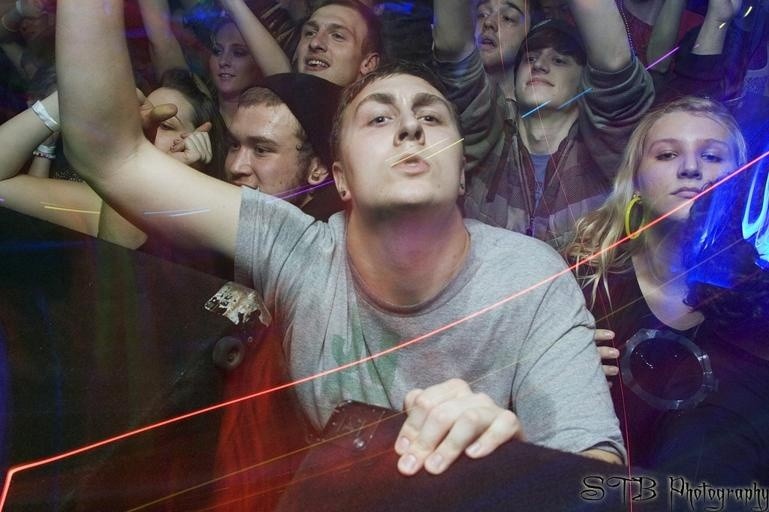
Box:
[[17, 0, 24, 19], [31, 100, 61, 132], [32, 145, 57, 161], [2, 15, 18, 34]]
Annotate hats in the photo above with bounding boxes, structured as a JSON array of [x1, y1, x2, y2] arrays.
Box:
[[513, 18, 587, 69], [239, 69, 348, 165]]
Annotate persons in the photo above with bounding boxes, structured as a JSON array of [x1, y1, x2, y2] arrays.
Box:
[[294, 0, 385, 85], [677, 0, 768, 120], [0, 66, 227, 274], [472, 0, 534, 119], [21, 26, 54, 79], [26, 59, 57, 106], [433, 0, 657, 239], [55, 0, 629, 475], [98, 73, 344, 282], [139, 1, 211, 98], [208, 0, 293, 128], [557, 92, 769, 511], [0, 0, 55, 83], [645, 0, 685, 102]]
